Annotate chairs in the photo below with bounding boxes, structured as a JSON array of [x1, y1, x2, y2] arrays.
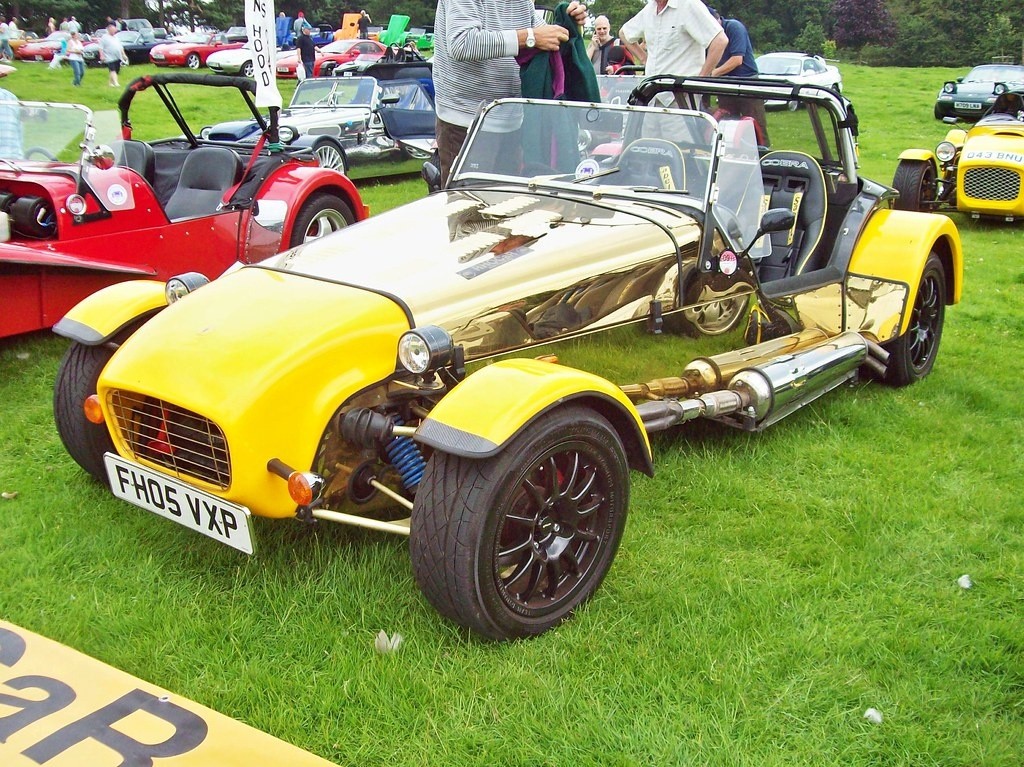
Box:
[[165, 147, 243, 220], [612, 137, 687, 189], [736, 149, 828, 284], [109, 139, 155, 187]]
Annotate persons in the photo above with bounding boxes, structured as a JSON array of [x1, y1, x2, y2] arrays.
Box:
[[66, 32, 85, 87], [50, 37, 69, 70], [355, 10, 372, 40], [431, 0, 587, 197], [619, 0, 729, 151], [106, 17, 116, 30], [585, 15, 636, 75], [48, 17, 56, 36], [0, 16, 20, 61], [296, 23, 324, 78], [118, 17, 130, 31], [293, 10, 313, 48], [98, 24, 129, 87], [707, 6, 770, 152], [60, 18, 69, 32], [166, 26, 181, 36], [276, 12, 292, 48], [68, 16, 82, 33]]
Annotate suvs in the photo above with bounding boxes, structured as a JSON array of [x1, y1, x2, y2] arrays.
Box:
[[95, 19, 153, 38]]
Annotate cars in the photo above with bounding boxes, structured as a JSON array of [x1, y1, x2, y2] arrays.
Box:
[[149, 33, 228, 70], [276, 38, 387, 78], [206, 41, 297, 75], [204, 25, 219, 34], [288, 23, 385, 48], [82, 28, 177, 65], [227, 26, 248, 41], [379, 13, 434, 50], [590, 63, 763, 164], [934, 63, 1024, 122], [0, 73, 367, 342], [0, 29, 40, 60], [755, 52, 843, 111], [15, 30, 82, 61], [202, 61, 440, 185], [332, 39, 425, 77], [892, 94, 1024, 223], [55, 75, 965, 638]]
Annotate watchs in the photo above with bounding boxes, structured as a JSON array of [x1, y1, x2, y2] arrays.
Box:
[[525, 27, 536, 49]]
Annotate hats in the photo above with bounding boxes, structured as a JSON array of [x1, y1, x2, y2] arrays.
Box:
[[707, 6, 715, 15], [106, 20, 115, 28], [302, 23, 311, 31], [298, 11, 304, 16]]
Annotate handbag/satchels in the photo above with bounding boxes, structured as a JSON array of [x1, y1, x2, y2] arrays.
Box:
[[45, 27, 50, 36], [296, 62, 306, 81]]
